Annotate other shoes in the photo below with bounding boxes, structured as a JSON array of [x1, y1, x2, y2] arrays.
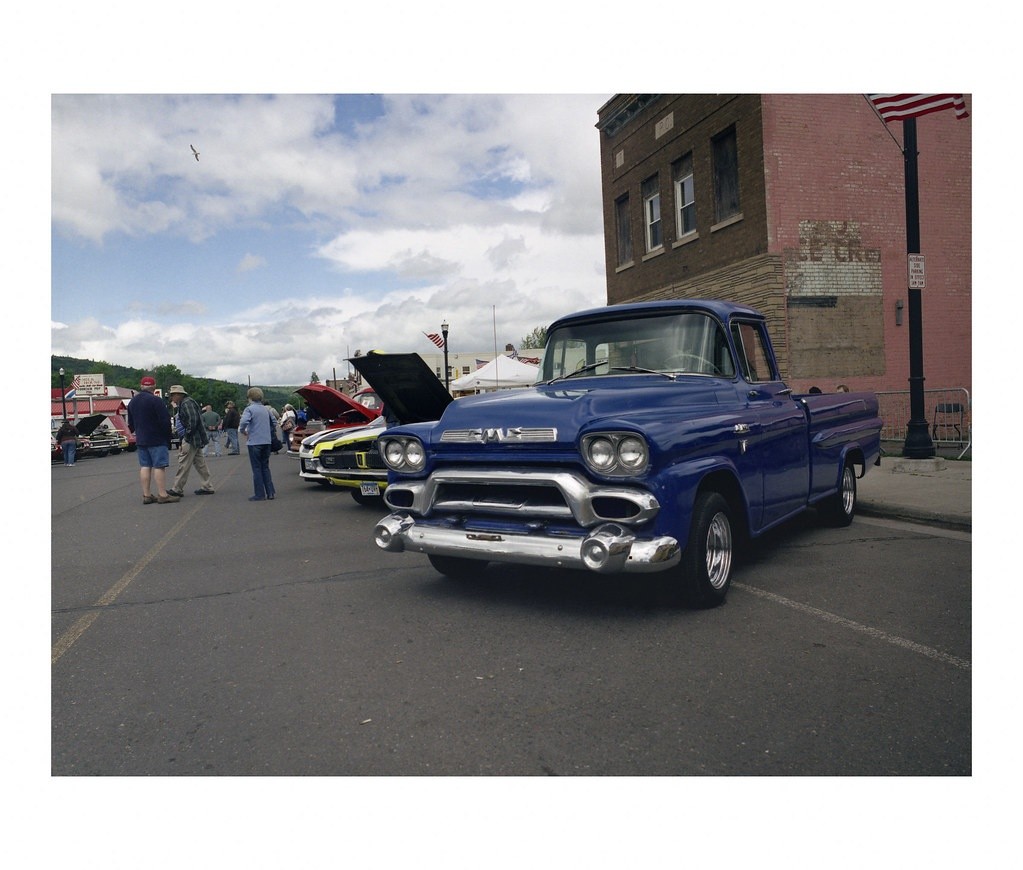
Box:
[[143, 493, 159, 503], [194, 488, 215, 495], [166, 489, 184, 497], [67, 463, 76, 467], [157, 493, 181, 504], [248, 495, 267, 501], [268, 495, 274, 500], [228, 452, 239, 455]]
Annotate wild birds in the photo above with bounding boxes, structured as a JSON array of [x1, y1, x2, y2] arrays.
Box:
[[190, 144, 200, 162]]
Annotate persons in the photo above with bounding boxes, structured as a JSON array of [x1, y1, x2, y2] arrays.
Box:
[[280, 403, 295, 452], [265, 402, 279, 455], [238, 387, 276, 501], [304, 400, 313, 418], [127, 377, 180, 503], [224, 405, 231, 448], [166, 385, 214, 497], [225, 401, 240, 455], [297, 406, 307, 422], [56, 420, 80, 466], [201, 405, 222, 457]]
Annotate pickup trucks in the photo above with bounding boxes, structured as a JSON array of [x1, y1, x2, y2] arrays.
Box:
[[372, 298, 891, 609]]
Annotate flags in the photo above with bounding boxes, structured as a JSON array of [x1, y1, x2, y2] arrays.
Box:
[[428, 334, 444, 348]]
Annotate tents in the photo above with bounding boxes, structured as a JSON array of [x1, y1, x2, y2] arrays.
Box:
[[451, 354, 543, 397]]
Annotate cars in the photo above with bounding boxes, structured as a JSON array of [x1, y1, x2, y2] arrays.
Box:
[[287, 351, 458, 512], [51, 413, 129, 460]]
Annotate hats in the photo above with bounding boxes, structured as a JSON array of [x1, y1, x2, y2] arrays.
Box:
[[170, 385, 187, 394], [141, 378, 156, 386], [61, 419, 70, 424]]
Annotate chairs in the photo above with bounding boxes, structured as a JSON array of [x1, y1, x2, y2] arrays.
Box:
[[930, 403, 964, 451]]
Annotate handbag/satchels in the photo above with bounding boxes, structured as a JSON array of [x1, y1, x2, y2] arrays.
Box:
[[270, 430, 283, 452], [282, 418, 293, 430]]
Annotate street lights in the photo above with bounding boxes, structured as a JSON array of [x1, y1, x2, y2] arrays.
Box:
[[438, 317, 451, 396], [59, 367, 70, 424]]
